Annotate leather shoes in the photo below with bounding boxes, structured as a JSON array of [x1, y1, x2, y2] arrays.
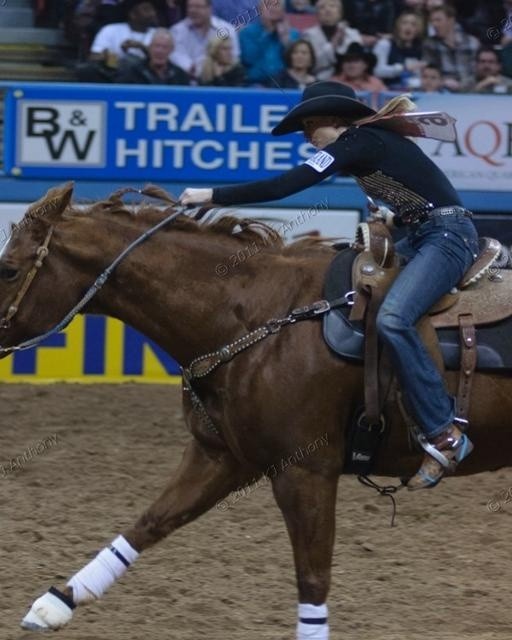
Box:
[[407, 423, 474, 492]]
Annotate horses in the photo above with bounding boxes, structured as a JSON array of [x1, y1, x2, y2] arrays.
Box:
[[0, 175, 512, 640]]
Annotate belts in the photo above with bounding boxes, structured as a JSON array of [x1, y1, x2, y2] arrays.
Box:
[[428, 205, 474, 220]]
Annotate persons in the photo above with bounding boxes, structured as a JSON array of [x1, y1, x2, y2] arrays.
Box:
[[177, 80, 480, 492], [32, 0, 511, 94]]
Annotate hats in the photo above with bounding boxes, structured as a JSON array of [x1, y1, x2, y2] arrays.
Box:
[[335, 43, 376, 68], [271, 81, 379, 136]]
